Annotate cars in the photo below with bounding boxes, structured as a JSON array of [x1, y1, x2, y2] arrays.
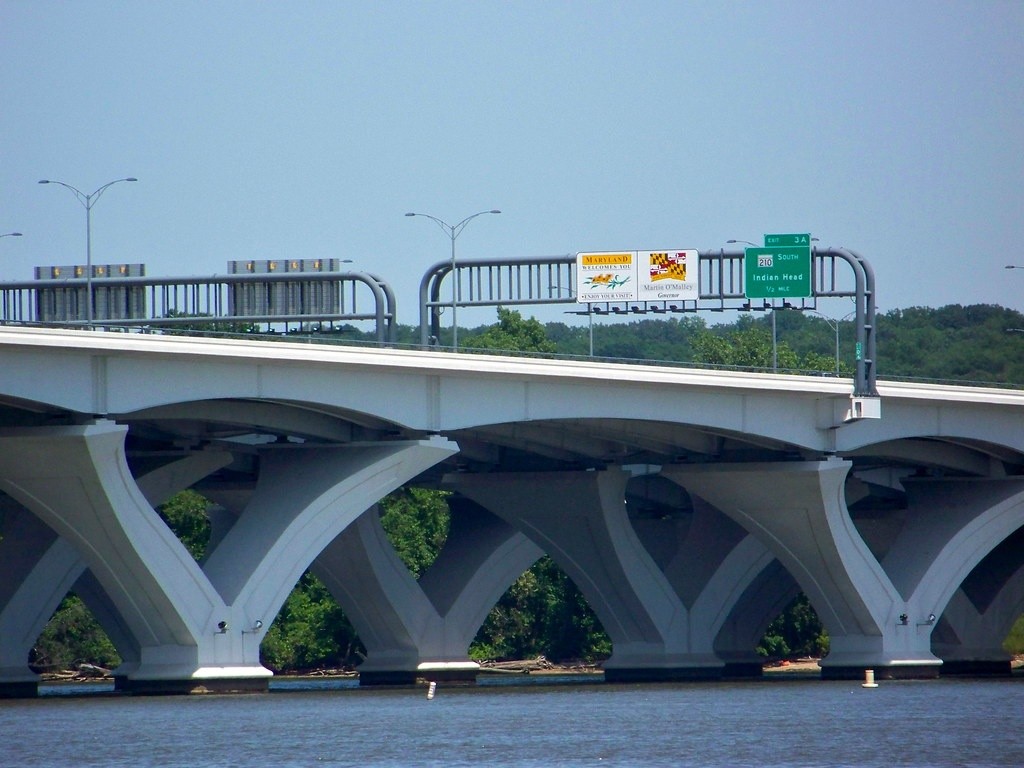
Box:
[[808, 370, 837, 377]]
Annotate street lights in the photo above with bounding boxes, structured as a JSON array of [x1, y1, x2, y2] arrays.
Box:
[[1004, 265, 1024, 269], [726, 237, 819, 374], [405, 210, 502, 353], [0, 233, 23, 237], [812, 306, 879, 378], [307, 260, 354, 344], [38, 178, 138, 331], [1006, 329, 1024, 332], [548, 286, 593, 356]]
